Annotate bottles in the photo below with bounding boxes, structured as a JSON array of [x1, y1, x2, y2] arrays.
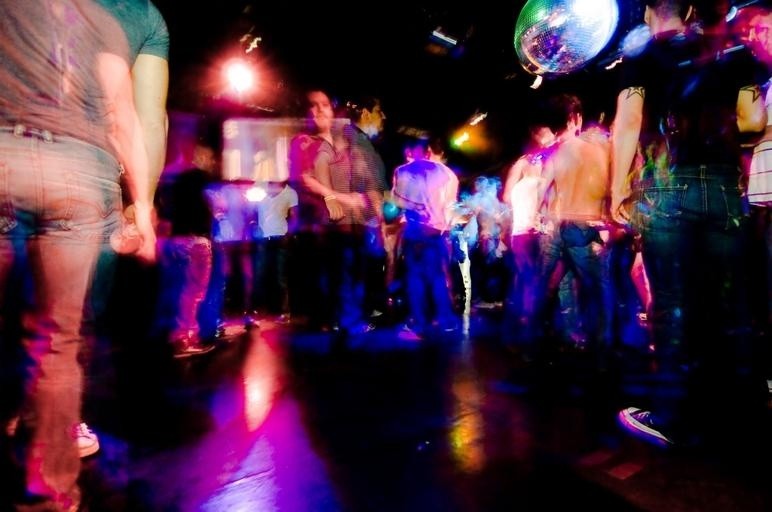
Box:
[[109, 228, 143, 256]]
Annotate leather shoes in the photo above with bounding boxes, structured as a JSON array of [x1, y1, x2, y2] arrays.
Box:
[[175, 335, 214, 358]]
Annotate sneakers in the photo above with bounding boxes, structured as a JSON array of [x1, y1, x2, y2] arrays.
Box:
[[73, 421, 100, 459], [619, 402, 681, 445]]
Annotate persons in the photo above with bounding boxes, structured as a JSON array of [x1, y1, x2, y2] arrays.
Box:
[[1, 1, 771, 510]]
